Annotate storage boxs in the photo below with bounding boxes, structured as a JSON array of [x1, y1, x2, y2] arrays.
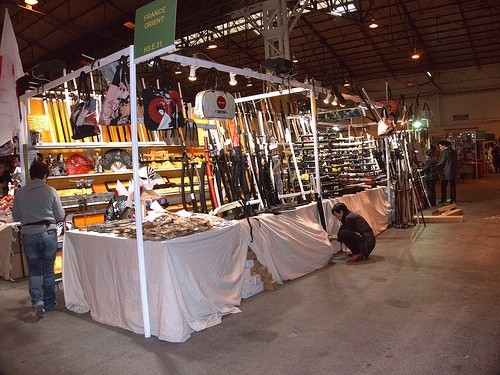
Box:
[[241, 249, 279, 300]]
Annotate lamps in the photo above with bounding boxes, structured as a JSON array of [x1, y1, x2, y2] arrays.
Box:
[[188, 64, 198, 82], [411, 47, 420, 59], [229, 72, 238, 86], [323, 91, 333, 104], [369, 16, 379, 30], [246, 78, 252, 88], [24, 0, 38, 5], [206, 37, 218, 49], [332, 95, 338, 106]]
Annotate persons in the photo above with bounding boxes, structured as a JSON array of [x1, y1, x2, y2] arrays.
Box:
[[0, 170, 10, 195], [421, 149, 436, 206], [436, 140, 457, 204], [493, 143, 500, 173], [33, 153, 66, 172], [327, 202, 376, 262], [11, 162, 65, 316]]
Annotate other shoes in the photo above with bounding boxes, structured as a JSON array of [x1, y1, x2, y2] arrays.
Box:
[[438, 201, 447, 205], [45, 301, 58, 311], [450, 200, 456, 205], [431, 202, 436, 206], [348, 253, 353, 256], [34, 303, 46, 316], [346, 252, 363, 263]]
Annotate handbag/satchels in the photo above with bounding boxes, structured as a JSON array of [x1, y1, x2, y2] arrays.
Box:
[[194, 69, 235, 119], [141, 56, 184, 131], [70, 71, 102, 139], [99, 56, 130, 126]]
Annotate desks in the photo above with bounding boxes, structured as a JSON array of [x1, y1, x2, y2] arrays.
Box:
[[62, 214, 242, 346], [240, 184, 390, 281], [461, 162, 481, 184]]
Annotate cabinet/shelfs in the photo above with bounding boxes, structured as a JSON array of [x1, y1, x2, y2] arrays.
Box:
[[21, 142, 222, 283]]
